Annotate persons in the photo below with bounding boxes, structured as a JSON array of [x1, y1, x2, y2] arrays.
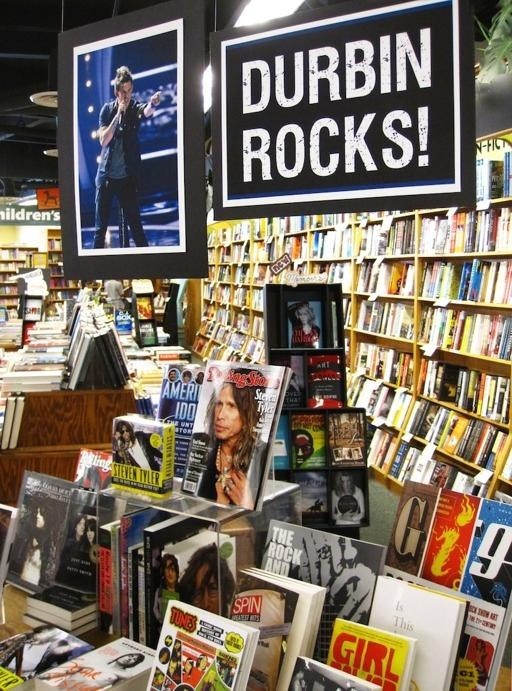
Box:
[[93, 65, 162, 249]]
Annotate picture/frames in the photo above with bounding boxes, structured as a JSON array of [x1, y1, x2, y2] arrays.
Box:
[[53, 0, 211, 286], [205, 0, 477, 218]]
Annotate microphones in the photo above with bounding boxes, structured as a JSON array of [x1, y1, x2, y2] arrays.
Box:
[[119, 112, 125, 125]]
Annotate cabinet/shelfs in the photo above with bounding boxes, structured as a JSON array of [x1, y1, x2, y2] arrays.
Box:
[[30, 226, 127, 321], [154, 283, 180, 345], [129, 280, 159, 349], [348, 206, 419, 488], [390, 192, 511, 521], [1, 241, 32, 343], [16, 264, 51, 348], [0, 302, 200, 504], [263, 277, 374, 538], [276, 205, 356, 425], [0, 480, 512, 691], [192, 218, 277, 382]]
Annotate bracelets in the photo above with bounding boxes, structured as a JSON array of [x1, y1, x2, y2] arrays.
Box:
[[150, 103, 158, 110]]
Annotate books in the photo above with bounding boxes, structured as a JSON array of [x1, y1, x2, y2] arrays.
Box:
[[2, 150, 510, 691]]
